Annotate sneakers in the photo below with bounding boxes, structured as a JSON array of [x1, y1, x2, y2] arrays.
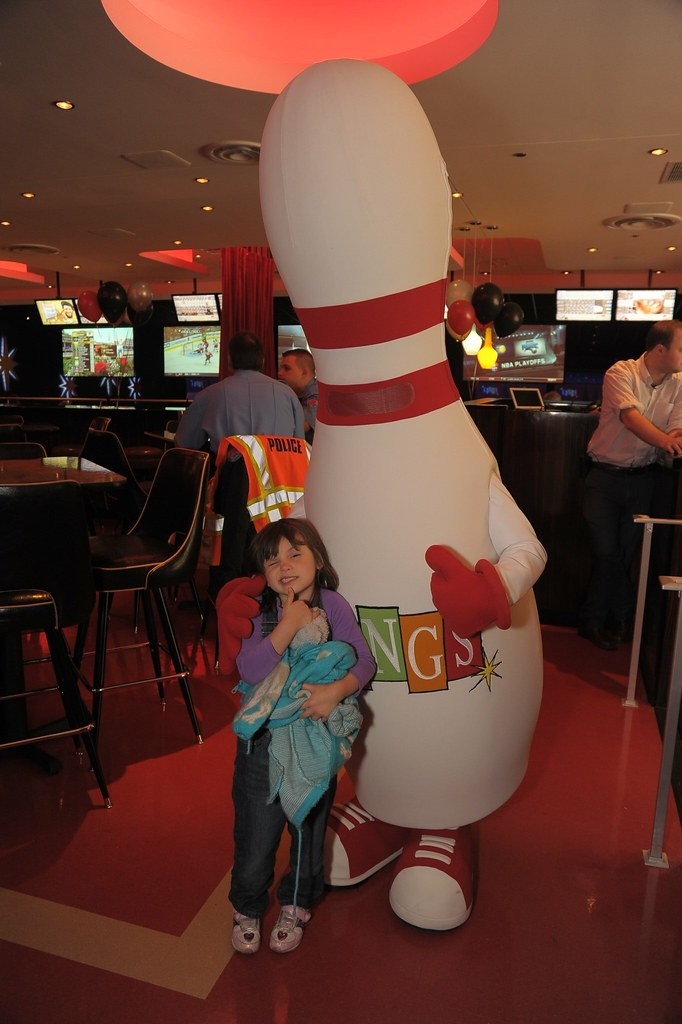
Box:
[[269, 905, 312, 953], [230, 907, 260, 954]]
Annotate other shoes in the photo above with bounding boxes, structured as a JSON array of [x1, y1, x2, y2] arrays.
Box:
[[578, 623, 616, 650]]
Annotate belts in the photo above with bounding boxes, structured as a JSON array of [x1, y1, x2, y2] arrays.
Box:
[[595, 460, 650, 476]]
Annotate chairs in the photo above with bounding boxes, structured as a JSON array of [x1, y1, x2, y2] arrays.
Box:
[[0, 412, 311, 810]]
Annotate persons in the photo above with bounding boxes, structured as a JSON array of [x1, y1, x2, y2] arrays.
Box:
[[542, 390, 562, 401], [172, 331, 305, 603], [227, 511, 379, 954], [60, 302, 76, 323], [192, 340, 219, 365], [278, 348, 318, 446], [577, 320, 682, 640]]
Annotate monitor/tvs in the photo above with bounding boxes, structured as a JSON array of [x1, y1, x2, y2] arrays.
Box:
[[462, 287, 677, 384], [34, 292, 317, 381]]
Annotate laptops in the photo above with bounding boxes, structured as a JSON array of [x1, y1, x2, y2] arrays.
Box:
[[510, 388, 544, 410]]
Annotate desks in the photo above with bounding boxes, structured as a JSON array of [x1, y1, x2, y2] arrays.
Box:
[[66, 405, 136, 412], [0, 456, 129, 774]]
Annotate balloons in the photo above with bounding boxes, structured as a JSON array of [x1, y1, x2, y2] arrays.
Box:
[[127, 302, 153, 328], [101, 307, 125, 327], [97, 280, 127, 323], [78, 290, 102, 325], [126, 280, 153, 311], [122, 306, 132, 324], [445, 279, 524, 341]]
